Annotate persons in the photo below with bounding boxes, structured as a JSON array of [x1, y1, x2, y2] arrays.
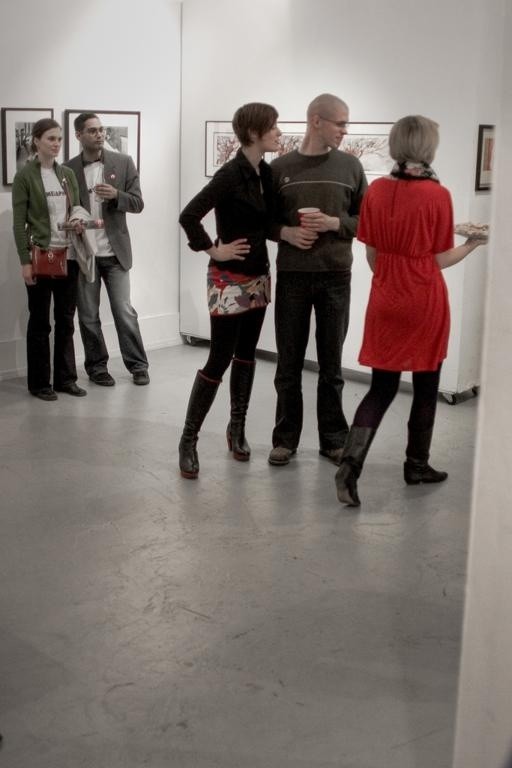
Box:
[[62, 113, 151, 387], [10, 117, 88, 402], [177, 101, 286, 480], [268, 92, 369, 465], [334, 115, 488, 508]]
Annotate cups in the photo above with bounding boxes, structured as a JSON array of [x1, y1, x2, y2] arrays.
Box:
[[298, 207, 320, 229]]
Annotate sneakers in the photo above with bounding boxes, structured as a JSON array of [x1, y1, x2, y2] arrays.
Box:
[[89, 372, 115, 386], [269, 446, 297, 465], [320, 449, 344, 464], [133, 369, 150, 385]]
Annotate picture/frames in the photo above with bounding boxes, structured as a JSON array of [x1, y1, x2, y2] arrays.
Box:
[[204, 120, 397, 177], [1, 107, 53, 185], [475, 124, 495, 191], [64, 109, 140, 175]]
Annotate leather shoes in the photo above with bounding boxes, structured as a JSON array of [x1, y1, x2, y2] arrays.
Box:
[[53, 383, 87, 396], [29, 388, 57, 400]]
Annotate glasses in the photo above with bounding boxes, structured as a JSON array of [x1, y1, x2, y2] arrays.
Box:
[[320, 116, 348, 127]]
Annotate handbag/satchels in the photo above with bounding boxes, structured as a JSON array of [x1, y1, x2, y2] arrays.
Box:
[[31, 245, 68, 276]]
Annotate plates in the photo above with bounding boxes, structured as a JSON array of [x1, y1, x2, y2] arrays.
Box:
[[454, 223, 488, 240]]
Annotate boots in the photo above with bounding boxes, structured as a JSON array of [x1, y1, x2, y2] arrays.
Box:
[[179, 369, 222, 479], [226, 356, 256, 461], [405, 422, 448, 484], [336, 425, 376, 506]]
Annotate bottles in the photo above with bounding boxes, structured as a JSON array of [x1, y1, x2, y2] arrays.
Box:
[[95, 164, 106, 202]]
